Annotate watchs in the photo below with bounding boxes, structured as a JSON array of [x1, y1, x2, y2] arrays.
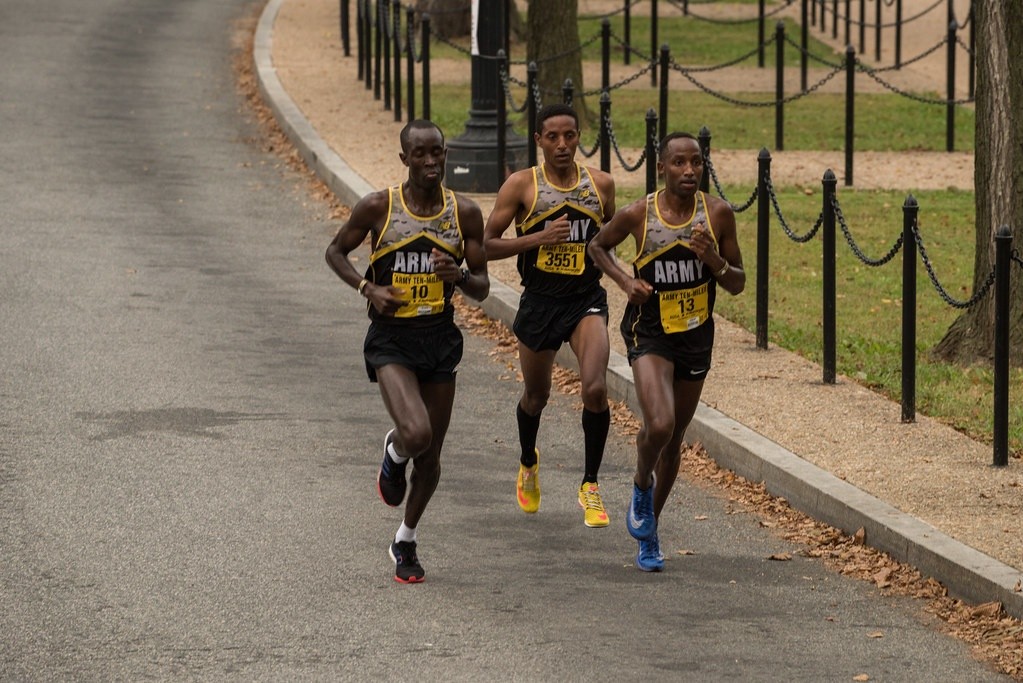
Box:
[[462, 267, 470, 280], [713, 260, 730, 278]]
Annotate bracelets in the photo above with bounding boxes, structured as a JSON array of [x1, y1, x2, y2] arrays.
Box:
[[357, 280, 367, 295]]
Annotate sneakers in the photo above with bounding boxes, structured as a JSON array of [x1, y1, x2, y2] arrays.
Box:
[[377, 428, 410, 507], [577, 482, 609, 528], [389, 535, 425, 583], [636, 526, 665, 572], [626, 468, 656, 540], [517, 447, 541, 512]]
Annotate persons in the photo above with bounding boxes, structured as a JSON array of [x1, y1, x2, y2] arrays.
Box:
[[482, 103, 616, 528], [325, 118, 491, 584], [587, 131, 747, 572]]
